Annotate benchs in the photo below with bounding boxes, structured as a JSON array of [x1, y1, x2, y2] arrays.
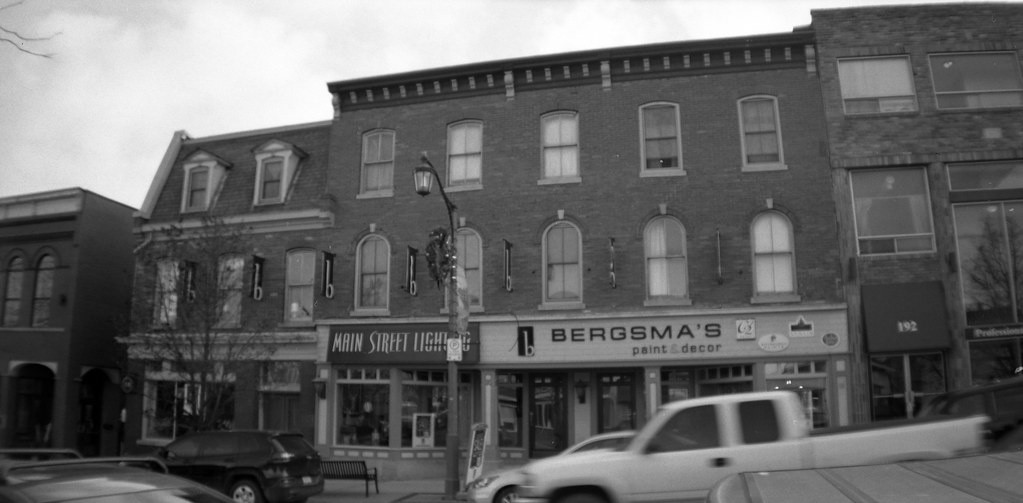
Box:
[[320, 459, 378, 497]]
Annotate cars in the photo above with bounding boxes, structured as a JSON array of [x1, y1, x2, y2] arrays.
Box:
[[459, 430, 700, 503]]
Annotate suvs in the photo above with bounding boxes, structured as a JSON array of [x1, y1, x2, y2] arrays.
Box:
[[914, 375, 1023, 452], [1, 448, 240, 503], [119, 428, 324, 503]]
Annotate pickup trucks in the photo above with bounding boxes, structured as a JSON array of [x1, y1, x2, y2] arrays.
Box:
[[515, 389, 989, 503]]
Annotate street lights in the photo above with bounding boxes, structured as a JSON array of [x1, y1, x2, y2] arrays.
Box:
[[412, 152, 460, 498]]
